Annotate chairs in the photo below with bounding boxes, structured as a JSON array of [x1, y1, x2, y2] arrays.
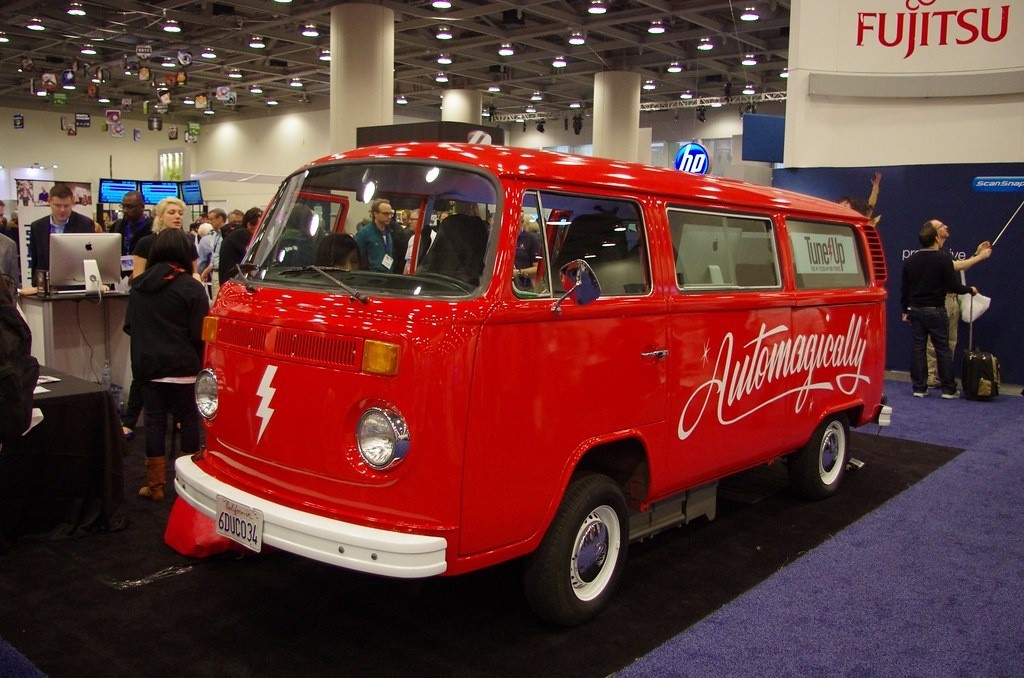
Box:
[[551, 212, 627, 297], [416, 214, 490, 292]]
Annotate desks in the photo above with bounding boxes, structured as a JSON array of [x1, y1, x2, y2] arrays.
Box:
[[23, 364, 132, 541]]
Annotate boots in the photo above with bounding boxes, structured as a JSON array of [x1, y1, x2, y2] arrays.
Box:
[[180, 447, 202, 456], [139, 455, 166, 502]]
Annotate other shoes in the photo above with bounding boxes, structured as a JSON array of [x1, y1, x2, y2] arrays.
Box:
[[121, 426, 135, 440], [928, 385, 937, 390]]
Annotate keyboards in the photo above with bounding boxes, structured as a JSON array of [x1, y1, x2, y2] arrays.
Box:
[[54, 290, 124, 294]]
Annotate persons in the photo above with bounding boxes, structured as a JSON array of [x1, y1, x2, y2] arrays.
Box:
[[901, 220, 991, 399], [17, 180, 51, 206], [837, 172, 881, 228], [273, 197, 561, 293], [0, 185, 263, 501]]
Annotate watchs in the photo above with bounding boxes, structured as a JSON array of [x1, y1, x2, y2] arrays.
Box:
[[518, 268, 522, 278]]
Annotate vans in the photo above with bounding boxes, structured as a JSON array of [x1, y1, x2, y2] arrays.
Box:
[[172, 140, 891, 630]]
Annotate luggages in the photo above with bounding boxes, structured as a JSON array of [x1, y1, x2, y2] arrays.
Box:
[[961, 292, 1000, 401]]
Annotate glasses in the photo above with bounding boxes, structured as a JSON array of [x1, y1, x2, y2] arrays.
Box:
[[936, 223, 944, 231], [379, 211, 394, 216], [119, 203, 142, 209], [410, 218, 419, 221]]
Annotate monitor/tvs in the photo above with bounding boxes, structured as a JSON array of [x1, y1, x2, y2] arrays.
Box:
[[49, 233, 122, 295], [98, 180, 204, 205]]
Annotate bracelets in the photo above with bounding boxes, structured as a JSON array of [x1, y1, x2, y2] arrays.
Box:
[[972, 253, 977, 257]]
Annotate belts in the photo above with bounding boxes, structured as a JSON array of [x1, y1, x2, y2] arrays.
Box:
[[911, 306, 936, 310]]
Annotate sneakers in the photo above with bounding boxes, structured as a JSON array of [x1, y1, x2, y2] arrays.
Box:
[[942, 390, 960, 399], [913, 389, 929, 397]]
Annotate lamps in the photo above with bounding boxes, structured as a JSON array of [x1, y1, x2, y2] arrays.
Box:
[[0, 0, 788, 123]]
